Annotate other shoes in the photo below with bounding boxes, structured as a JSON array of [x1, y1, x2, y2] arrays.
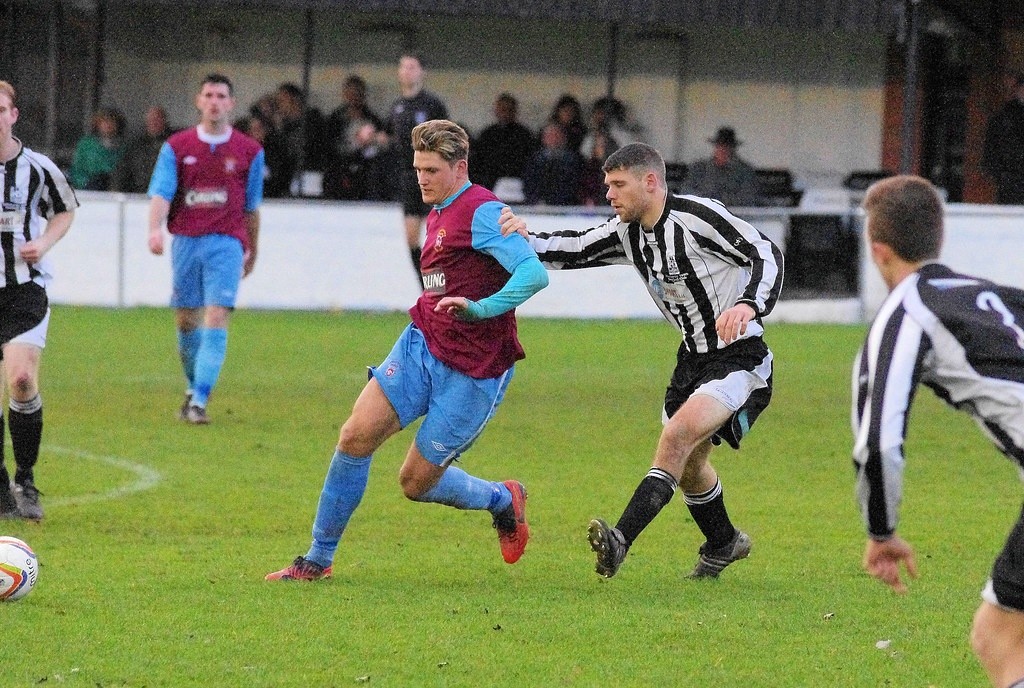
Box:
[[181, 393, 207, 424]]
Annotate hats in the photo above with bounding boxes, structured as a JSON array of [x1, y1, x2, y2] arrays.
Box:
[[707, 128, 743, 145]]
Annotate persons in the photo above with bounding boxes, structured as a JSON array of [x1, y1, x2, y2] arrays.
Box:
[[64, 75, 648, 207], [0, 80, 81, 524], [495, 142, 775, 584], [678, 127, 758, 208], [146, 73, 265, 425], [264, 118, 547, 585], [848, 176, 1024, 688], [387, 51, 447, 291]]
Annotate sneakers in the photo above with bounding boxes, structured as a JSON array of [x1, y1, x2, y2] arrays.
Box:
[[265, 556, 332, 582], [491, 479, 530, 564], [0, 473, 45, 519], [683, 524, 751, 580], [587, 518, 627, 578]]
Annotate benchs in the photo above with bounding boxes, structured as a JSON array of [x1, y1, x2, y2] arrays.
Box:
[[661, 162, 802, 208]]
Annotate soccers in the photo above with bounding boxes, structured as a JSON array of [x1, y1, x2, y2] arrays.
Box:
[[0, 536, 38, 602]]
